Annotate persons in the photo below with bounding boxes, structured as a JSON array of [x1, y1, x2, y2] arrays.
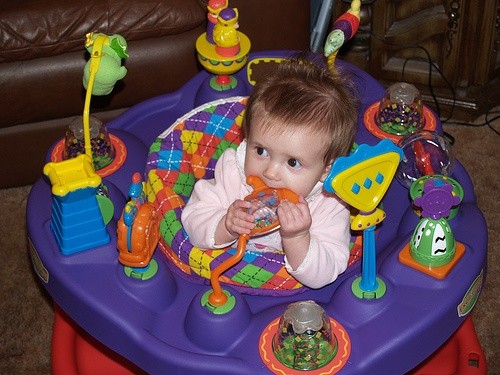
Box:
[[180, 54, 358, 289]]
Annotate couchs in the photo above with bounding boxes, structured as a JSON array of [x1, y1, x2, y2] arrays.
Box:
[[0, 0, 312, 189]]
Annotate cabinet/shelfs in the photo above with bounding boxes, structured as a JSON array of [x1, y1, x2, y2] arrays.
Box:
[[332, 0, 500, 121]]
